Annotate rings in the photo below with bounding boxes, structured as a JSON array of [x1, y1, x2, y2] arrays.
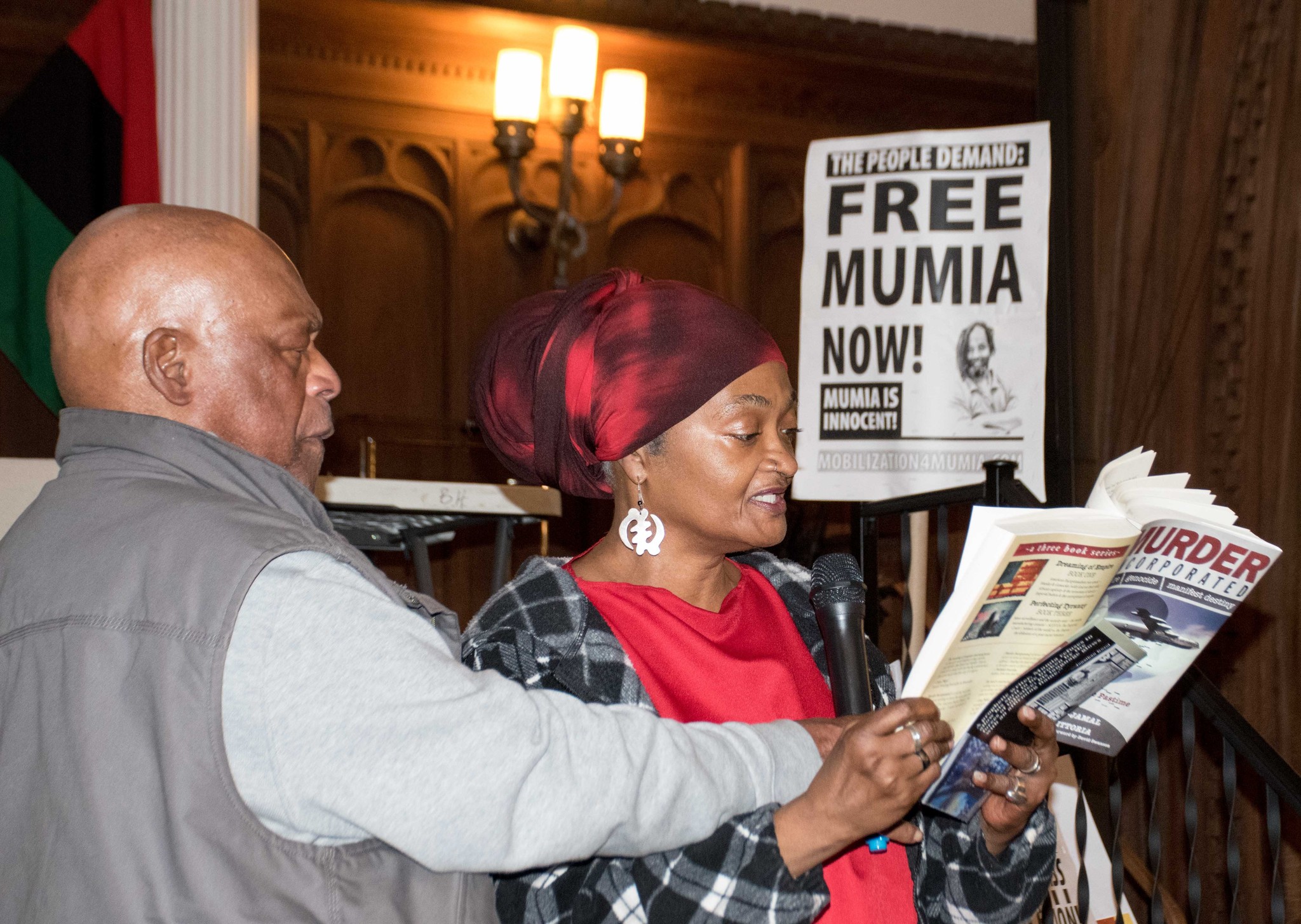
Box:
[[1013, 747, 1041, 775], [908, 725, 922, 754], [917, 750, 931, 770], [1005, 775, 1027, 805]]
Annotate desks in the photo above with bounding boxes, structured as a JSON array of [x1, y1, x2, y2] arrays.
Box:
[[0, 458, 566, 621]]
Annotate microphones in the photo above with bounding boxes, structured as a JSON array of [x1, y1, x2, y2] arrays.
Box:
[[809, 553, 889, 852]]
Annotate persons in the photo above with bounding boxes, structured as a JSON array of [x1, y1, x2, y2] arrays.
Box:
[[0, 203, 923, 924], [461, 268, 1058, 924], [978, 611, 999, 639]]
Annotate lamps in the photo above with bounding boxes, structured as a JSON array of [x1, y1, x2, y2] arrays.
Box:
[[490, 24, 649, 292]]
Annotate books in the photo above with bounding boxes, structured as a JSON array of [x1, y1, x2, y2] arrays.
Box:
[[896, 446, 1281, 765]]
